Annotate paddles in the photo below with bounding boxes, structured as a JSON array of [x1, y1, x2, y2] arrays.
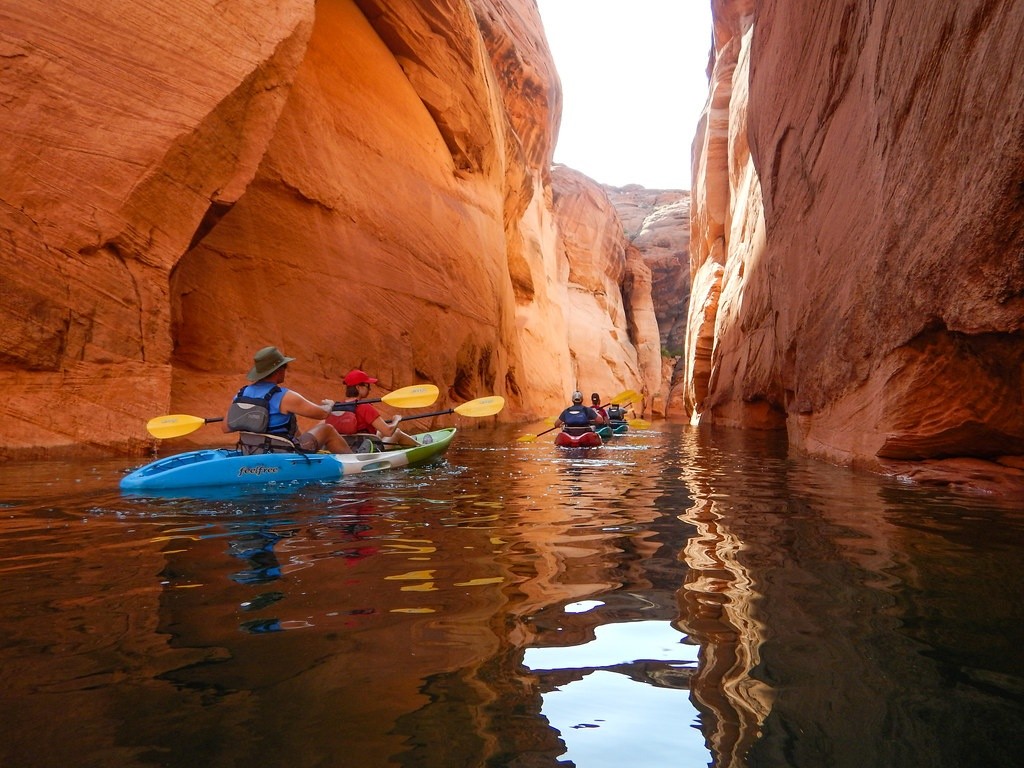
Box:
[[387, 397, 507, 421], [516, 388, 637, 442], [624, 393, 645, 410], [147, 383, 441, 441], [608, 418, 654, 430]]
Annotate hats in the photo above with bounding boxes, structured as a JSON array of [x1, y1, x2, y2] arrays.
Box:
[[343, 370, 377, 385], [572, 391, 583, 402], [592, 394, 599, 403], [246, 347, 296, 381]]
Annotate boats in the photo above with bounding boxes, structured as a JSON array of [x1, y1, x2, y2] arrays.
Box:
[[594, 426, 615, 437], [114, 425, 463, 498], [610, 424, 631, 433], [554, 429, 603, 449]]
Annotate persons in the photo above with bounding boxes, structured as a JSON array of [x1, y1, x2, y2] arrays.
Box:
[[316, 369, 426, 450], [588, 392, 613, 429], [605, 403, 627, 422], [221, 345, 353, 455], [554, 391, 604, 437]]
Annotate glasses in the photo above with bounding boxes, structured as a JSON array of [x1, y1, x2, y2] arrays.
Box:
[[361, 384, 371, 389]]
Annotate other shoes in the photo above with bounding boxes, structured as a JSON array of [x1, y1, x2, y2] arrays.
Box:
[[422, 434, 432, 444], [354, 439, 374, 453], [412, 436, 417, 441]]
